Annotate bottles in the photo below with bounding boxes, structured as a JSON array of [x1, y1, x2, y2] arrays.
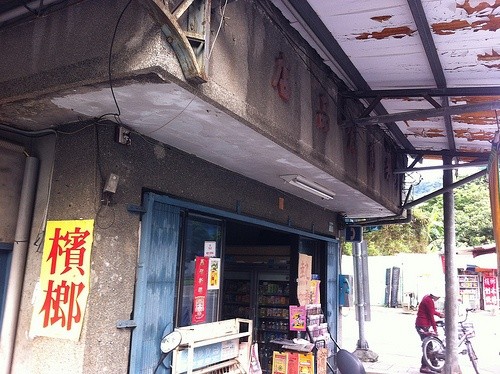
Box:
[[256, 320, 292, 374]]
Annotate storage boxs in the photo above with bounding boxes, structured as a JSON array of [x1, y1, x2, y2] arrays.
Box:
[[272, 350, 314, 374]]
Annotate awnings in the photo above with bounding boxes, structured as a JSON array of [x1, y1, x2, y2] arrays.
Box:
[[255, 0, 500, 210]]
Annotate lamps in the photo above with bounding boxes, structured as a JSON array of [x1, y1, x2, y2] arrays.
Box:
[[280, 174, 337, 201]]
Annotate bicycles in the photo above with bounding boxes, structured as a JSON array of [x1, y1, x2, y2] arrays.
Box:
[[421, 308, 480, 374]]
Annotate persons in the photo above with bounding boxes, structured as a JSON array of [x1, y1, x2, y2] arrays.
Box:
[[416, 292, 445, 374]]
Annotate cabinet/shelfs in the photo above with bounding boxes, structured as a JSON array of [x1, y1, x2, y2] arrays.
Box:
[[170, 318, 253, 374], [458, 274, 480, 310]]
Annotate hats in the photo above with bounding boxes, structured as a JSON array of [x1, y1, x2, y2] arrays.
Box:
[[429, 287, 445, 298]]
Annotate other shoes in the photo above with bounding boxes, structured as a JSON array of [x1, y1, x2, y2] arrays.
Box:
[[420, 366, 437, 374]]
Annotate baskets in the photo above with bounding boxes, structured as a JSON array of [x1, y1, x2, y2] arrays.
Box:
[[458, 323, 476, 341]]
